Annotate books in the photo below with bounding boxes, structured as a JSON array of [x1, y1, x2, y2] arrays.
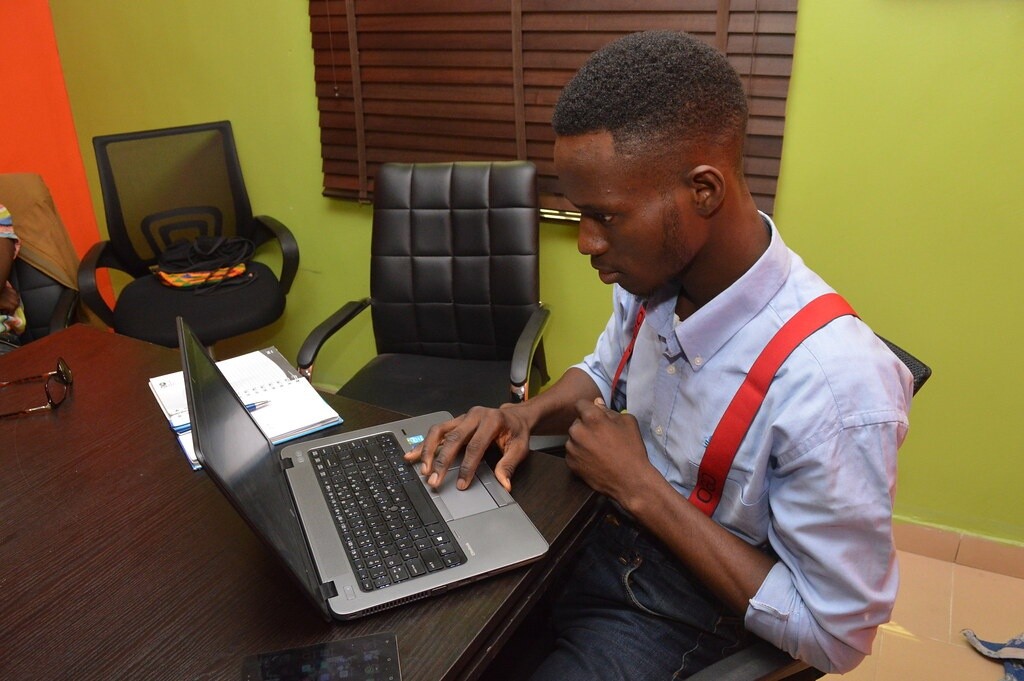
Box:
[[148, 345, 344, 471]]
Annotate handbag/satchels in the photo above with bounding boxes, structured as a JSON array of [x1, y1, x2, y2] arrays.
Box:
[[148, 235, 258, 297]]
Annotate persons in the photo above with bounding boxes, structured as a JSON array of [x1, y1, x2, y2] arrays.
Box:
[[405, 29, 915, 681], [0, 204, 26, 356]]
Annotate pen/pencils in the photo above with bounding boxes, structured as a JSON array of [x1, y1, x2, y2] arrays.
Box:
[[173, 400, 270, 433]]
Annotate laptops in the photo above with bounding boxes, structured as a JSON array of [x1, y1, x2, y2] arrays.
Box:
[[177, 316, 551, 620]]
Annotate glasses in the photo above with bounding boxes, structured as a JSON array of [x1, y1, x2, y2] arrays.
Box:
[[0, 357, 72, 417]]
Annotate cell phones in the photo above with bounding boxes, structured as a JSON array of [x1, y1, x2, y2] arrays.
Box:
[[241, 631, 405, 681]]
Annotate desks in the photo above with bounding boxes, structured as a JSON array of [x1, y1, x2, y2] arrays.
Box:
[[0, 324, 605, 681]]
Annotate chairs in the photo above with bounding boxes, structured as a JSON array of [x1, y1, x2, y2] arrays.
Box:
[[0, 172, 89, 355], [291, 162, 552, 420], [77, 119, 300, 363], [686, 332, 932, 681]]
